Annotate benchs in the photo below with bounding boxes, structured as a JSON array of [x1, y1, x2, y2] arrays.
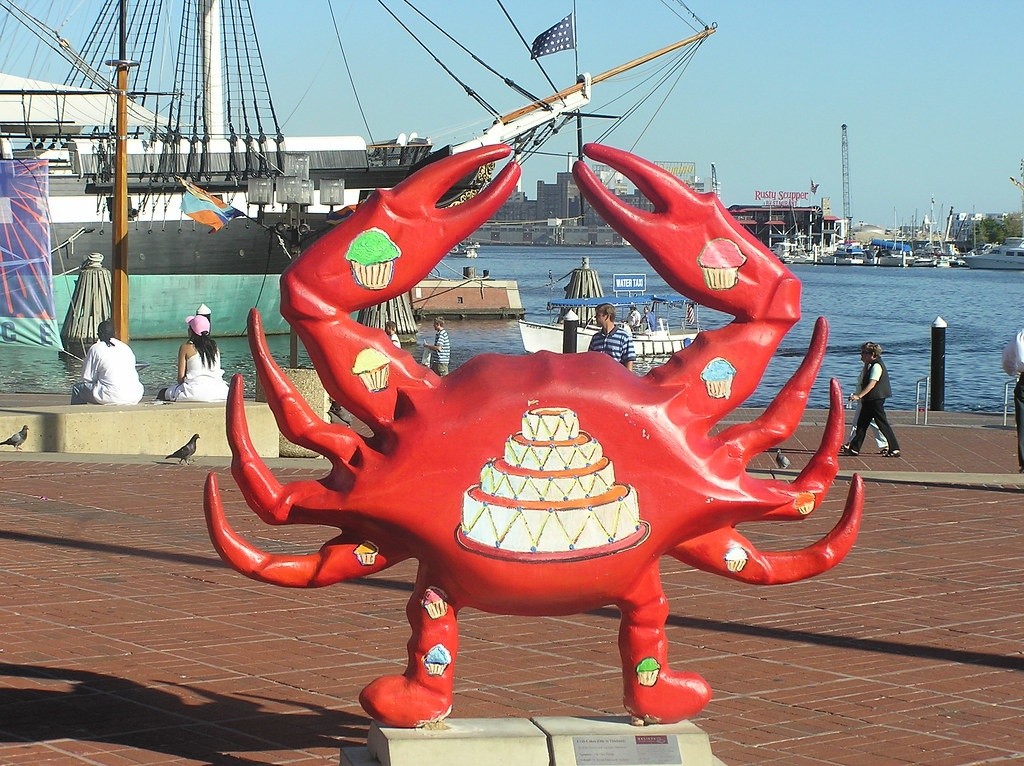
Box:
[[0, 396, 280, 459]]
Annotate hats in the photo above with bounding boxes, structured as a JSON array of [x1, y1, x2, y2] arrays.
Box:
[[184, 315, 211, 336]]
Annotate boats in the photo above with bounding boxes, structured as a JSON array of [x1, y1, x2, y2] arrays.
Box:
[[956, 235, 1024, 271], [762, 192, 971, 268], [516, 293, 710, 367]]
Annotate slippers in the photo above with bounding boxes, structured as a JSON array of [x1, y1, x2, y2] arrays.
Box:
[[882, 450, 900, 458], [838, 449, 859, 456]]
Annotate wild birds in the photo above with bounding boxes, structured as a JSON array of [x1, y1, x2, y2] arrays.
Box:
[[165, 433, 201, 465], [0, 425, 30, 452], [776, 449, 794, 471]]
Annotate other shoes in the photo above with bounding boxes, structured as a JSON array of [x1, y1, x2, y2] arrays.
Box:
[[840, 442, 851, 453], [874, 447, 889, 454]]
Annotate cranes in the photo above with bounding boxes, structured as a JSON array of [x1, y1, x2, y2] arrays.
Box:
[[841, 123, 852, 243]]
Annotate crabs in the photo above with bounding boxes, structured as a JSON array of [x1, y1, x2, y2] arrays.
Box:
[[205, 140, 863, 724]]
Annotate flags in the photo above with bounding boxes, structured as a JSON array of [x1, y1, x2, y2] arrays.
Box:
[[530, 11, 575, 60], [175, 181, 248, 234], [687, 304, 696, 325]]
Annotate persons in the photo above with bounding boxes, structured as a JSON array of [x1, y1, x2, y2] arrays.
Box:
[[423, 317, 450, 377], [1000, 326, 1024, 473], [155, 315, 231, 402], [838, 341, 901, 458], [69, 321, 145, 405], [587, 302, 636, 372], [621, 303, 656, 331], [385, 321, 401, 349]]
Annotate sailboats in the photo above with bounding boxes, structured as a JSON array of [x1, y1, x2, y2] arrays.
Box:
[[0, 0, 720, 352]]
